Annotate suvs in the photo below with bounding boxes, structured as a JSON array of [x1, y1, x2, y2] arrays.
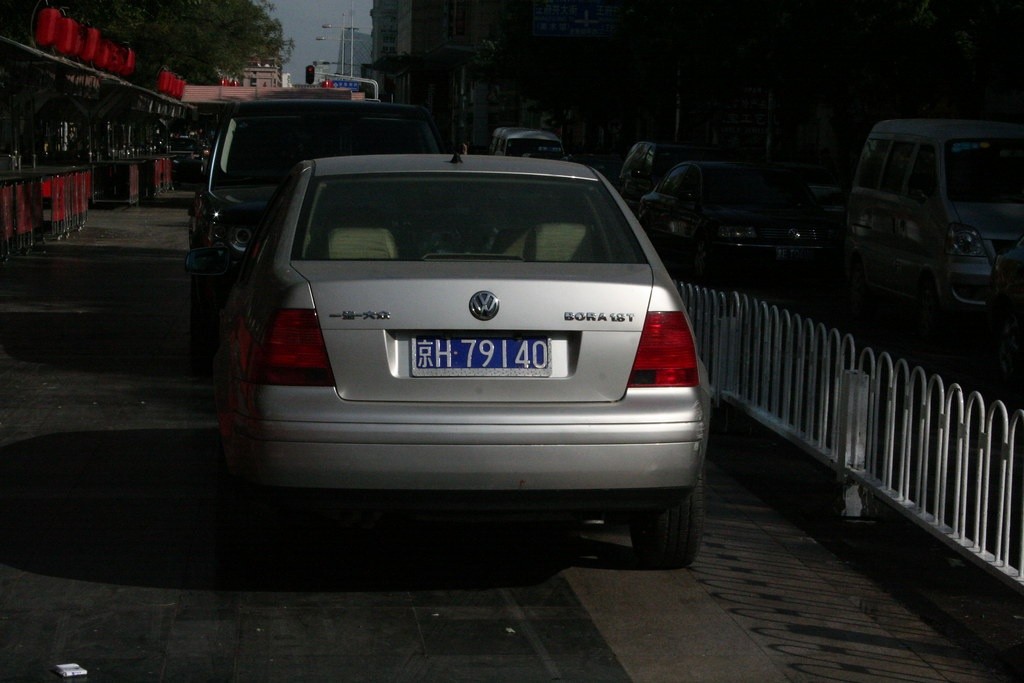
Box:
[[173, 96, 449, 344]]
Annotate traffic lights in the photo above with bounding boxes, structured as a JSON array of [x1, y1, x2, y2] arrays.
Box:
[[305, 65, 315, 84]]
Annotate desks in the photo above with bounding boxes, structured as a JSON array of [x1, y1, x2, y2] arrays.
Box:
[[93, 154, 173, 207], [0, 164, 92, 265]]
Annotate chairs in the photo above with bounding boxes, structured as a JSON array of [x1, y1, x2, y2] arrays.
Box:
[[323, 228, 399, 260], [491, 229, 527, 257], [524, 222, 595, 262]]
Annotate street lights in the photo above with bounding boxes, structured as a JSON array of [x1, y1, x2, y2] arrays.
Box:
[[316, 35, 363, 76], [321, 22, 359, 77]]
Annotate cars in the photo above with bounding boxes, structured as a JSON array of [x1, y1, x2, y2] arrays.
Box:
[[570, 153, 623, 194], [636, 161, 847, 284], [179, 148, 716, 575], [170, 135, 210, 180], [995, 228, 1024, 388], [793, 162, 846, 222]]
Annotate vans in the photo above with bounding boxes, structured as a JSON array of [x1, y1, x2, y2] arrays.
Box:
[[847, 118, 1024, 346], [619, 139, 718, 217], [488, 125, 567, 161]]
